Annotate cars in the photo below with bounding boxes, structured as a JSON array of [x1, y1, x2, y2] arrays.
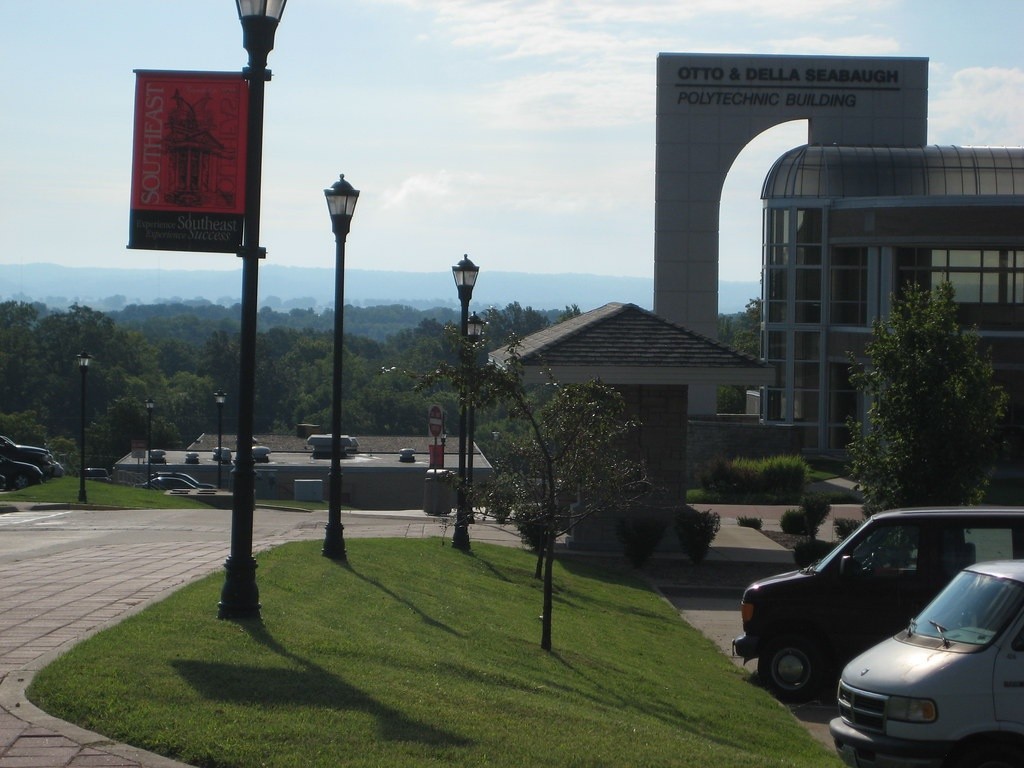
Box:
[[0, 435, 64, 491], [143, 472, 216, 489], [83, 467, 112, 483]]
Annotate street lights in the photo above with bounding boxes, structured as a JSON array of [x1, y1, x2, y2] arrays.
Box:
[[450, 253, 486, 553], [144, 397, 155, 490], [74, 347, 94, 504], [213, 388, 227, 489], [320, 172, 360, 558], [215, 1, 289, 616]]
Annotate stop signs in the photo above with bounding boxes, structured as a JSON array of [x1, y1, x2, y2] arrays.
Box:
[[429, 406, 442, 437]]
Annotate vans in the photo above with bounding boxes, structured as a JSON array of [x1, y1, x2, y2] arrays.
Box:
[[829, 560, 1023, 768], [730, 505, 1024, 702]]
